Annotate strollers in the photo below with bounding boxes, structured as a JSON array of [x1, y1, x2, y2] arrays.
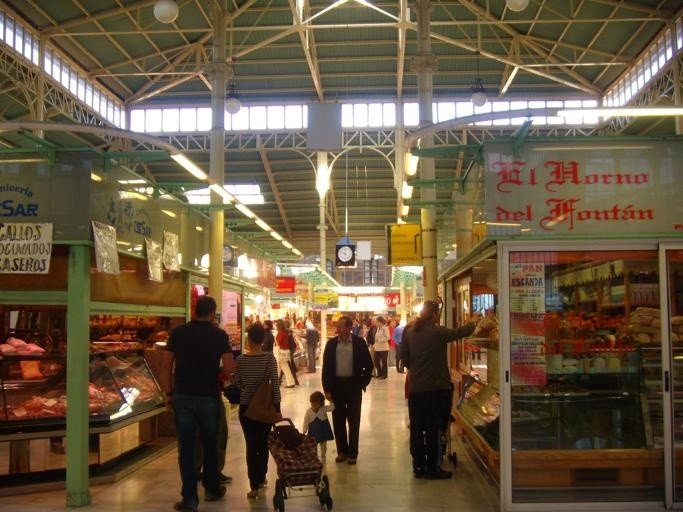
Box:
[[410, 380, 457, 468], [266, 417, 333, 512]]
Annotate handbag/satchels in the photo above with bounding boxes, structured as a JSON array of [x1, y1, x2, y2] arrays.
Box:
[[244, 358, 275, 425]]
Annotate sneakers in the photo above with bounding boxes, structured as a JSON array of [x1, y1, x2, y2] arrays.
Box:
[[247, 491, 258, 497], [304, 370, 315, 373], [174, 500, 197, 512], [396, 371, 401, 373], [194, 472, 203, 478], [400, 371, 406, 374], [376, 375, 381, 378], [335, 452, 348, 462], [414, 467, 427, 479], [423, 469, 452, 478], [216, 474, 233, 483], [284, 385, 294, 388], [204, 486, 226, 501], [259, 480, 268, 488], [347, 455, 357, 464], [380, 376, 386, 379]]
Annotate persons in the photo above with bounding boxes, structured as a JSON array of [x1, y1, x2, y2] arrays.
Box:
[[283, 319, 300, 386], [273, 319, 295, 388], [197, 319, 233, 485], [301, 322, 319, 373], [262, 320, 275, 355], [163, 296, 234, 512], [234, 321, 283, 500], [393, 319, 406, 374], [387, 319, 401, 366], [373, 317, 390, 380], [399, 300, 481, 479], [303, 392, 335, 469], [321, 316, 373, 465]]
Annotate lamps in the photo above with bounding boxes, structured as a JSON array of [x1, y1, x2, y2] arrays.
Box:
[[470, 51, 487, 107], [227, 61, 241, 115]]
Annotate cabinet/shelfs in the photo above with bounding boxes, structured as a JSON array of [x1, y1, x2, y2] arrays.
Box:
[[550, 262, 659, 326]]
